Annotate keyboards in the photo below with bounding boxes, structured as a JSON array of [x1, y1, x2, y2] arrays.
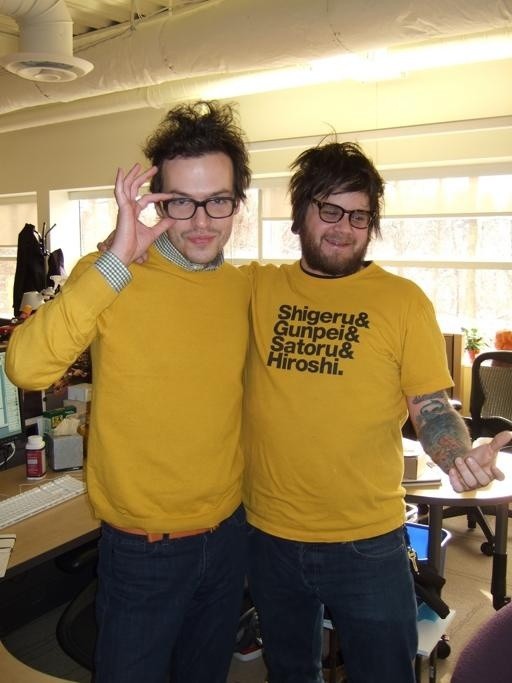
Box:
[[0, 474, 88, 527]]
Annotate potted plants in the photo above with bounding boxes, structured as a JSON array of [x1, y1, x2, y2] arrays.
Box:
[[460, 326, 484, 362]]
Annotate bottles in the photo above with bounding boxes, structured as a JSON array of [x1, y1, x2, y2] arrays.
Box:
[[25, 435, 45, 481]]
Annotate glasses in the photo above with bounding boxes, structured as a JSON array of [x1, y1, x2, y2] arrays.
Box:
[[160, 197, 237, 220], [311, 198, 373, 229]]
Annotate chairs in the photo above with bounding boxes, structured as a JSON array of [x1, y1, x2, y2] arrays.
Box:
[[454, 350, 512, 452]]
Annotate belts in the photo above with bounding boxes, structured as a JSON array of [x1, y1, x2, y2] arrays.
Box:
[[107, 523, 220, 543]]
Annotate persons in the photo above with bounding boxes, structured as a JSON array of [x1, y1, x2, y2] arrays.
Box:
[[4, 100, 251, 683], [97, 142, 512, 683]]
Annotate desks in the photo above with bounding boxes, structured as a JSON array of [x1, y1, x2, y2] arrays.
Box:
[[406, 440, 512, 609], [0, 407, 115, 682]]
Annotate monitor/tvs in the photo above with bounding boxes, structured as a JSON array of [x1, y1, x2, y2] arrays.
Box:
[[0, 347, 44, 449]]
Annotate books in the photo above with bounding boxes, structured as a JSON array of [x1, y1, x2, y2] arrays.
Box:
[[402, 464, 441, 486]]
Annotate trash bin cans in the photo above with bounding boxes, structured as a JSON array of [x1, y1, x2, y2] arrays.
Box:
[[404, 523, 453, 579]]
